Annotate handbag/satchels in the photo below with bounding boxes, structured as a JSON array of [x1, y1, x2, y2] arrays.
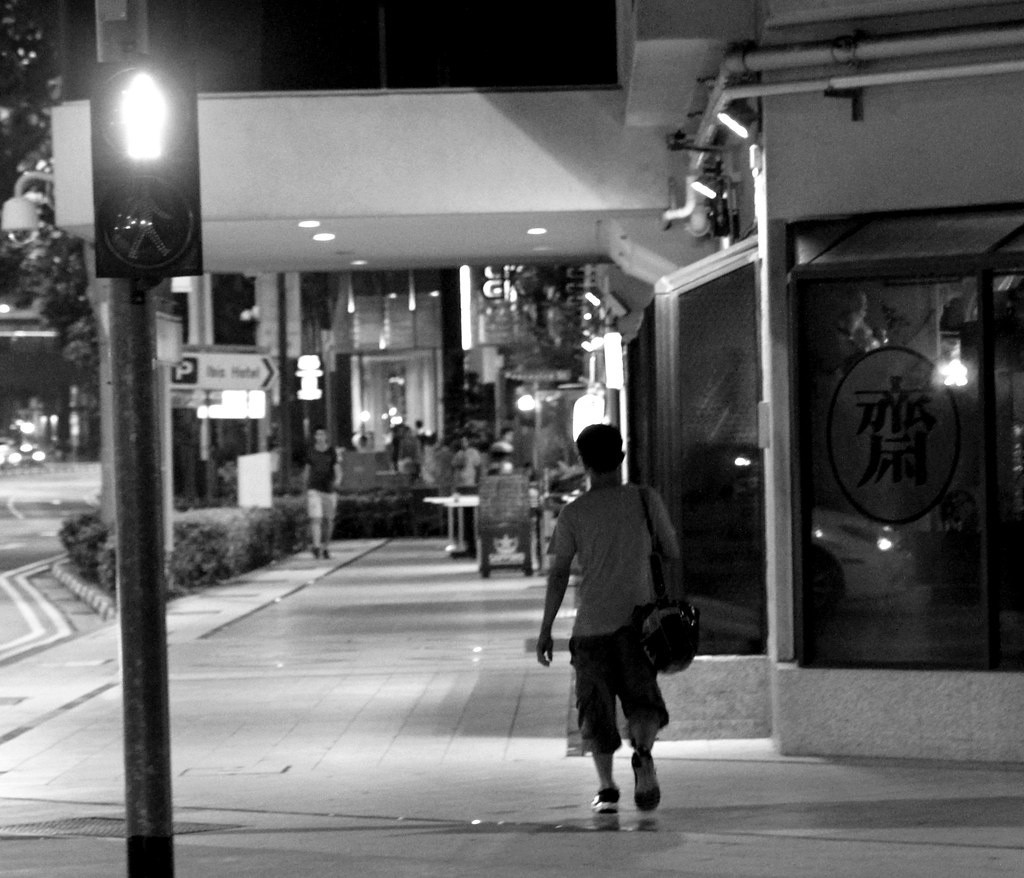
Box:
[[641, 600, 701, 675]]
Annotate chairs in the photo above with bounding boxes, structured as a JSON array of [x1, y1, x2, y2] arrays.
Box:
[[475, 474, 535, 578]]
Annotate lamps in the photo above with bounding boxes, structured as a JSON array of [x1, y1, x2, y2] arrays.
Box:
[[691, 176, 720, 199], [715, 102, 756, 139], [585, 289, 630, 316]]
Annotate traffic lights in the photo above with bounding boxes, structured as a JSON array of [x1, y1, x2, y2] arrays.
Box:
[[88, 56, 207, 281]]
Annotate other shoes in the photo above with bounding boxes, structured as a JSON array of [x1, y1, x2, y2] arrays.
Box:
[[312, 546, 330, 560], [591, 788, 620, 813], [632, 750, 660, 810]]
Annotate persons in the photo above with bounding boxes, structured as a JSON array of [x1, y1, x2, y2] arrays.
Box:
[[536, 424, 696, 813], [303, 428, 341, 557], [389, 420, 516, 558]]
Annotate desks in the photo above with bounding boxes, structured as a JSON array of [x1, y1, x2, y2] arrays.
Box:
[[426, 494, 543, 556]]
